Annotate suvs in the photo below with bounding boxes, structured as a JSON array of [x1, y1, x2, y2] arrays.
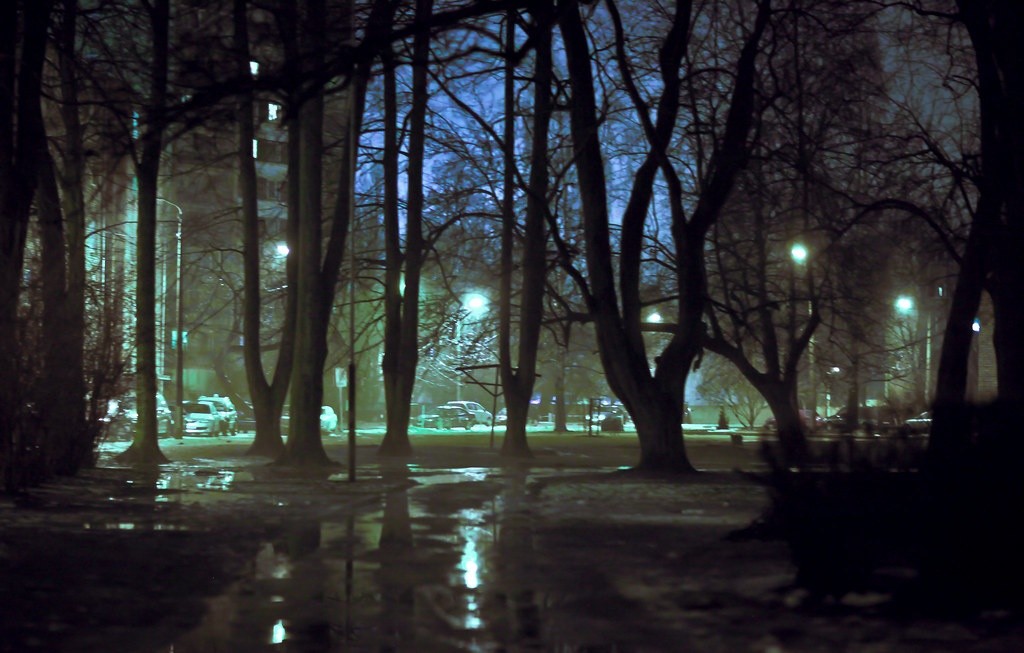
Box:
[[198, 394, 237, 436]]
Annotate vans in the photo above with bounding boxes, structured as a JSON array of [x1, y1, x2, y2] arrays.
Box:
[[446, 400, 493, 426]]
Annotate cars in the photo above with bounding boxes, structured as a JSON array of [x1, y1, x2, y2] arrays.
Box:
[[181, 400, 220, 437], [279, 405, 291, 430], [903, 411, 933, 433], [410, 402, 477, 430], [319, 405, 338, 433], [495, 407, 509, 425], [764, 404, 898, 435], [124, 392, 171, 437], [586, 405, 629, 426]]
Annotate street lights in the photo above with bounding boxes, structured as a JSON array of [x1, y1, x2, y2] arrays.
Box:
[[156, 197, 184, 440], [454, 295, 484, 401], [790, 243, 819, 435]]
